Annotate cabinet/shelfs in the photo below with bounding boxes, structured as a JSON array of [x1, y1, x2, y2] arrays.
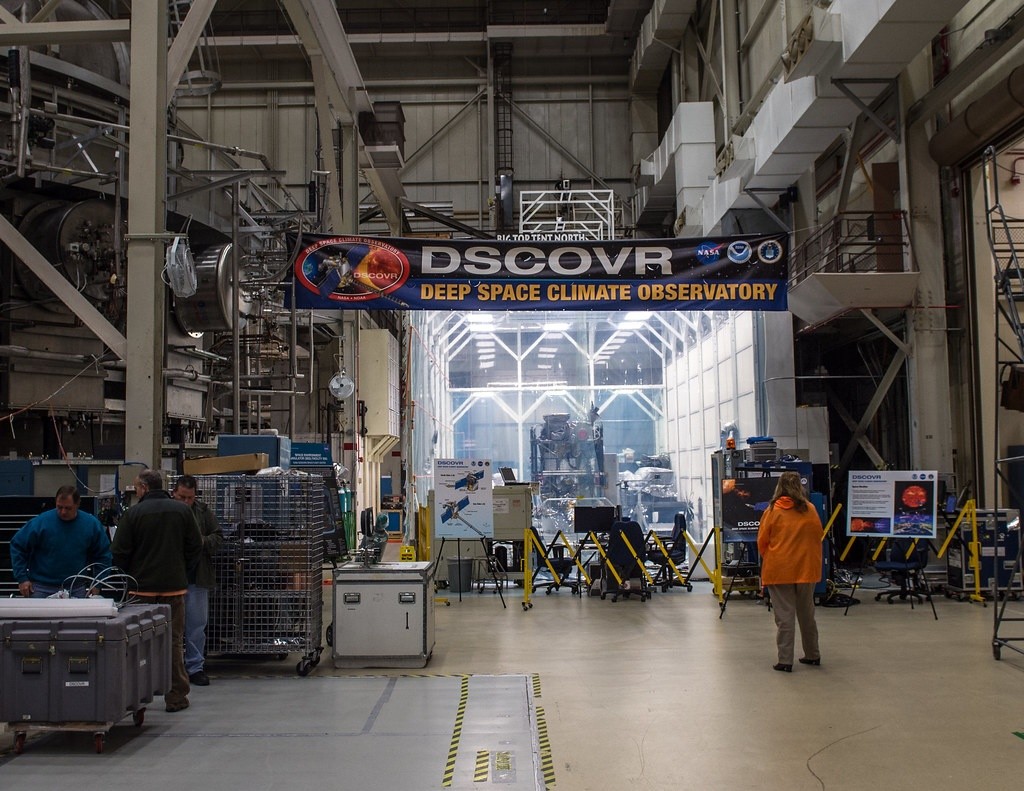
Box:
[[428, 484, 532, 594], [332, 561, 436, 669]]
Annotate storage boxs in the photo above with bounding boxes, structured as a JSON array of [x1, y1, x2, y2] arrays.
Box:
[[0, 601, 173, 723], [380, 508, 403, 535], [945, 507, 1024, 591]]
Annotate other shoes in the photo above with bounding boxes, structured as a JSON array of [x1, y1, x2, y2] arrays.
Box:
[[190, 671, 210, 686], [773, 663, 793, 672], [799, 659, 820, 666], [165, 696, 188, 712]]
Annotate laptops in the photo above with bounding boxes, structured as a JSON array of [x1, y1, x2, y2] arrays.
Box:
[[497, 467, 530, 485]]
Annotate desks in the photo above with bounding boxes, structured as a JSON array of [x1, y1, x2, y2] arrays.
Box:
[[573, 533, 658, 596]]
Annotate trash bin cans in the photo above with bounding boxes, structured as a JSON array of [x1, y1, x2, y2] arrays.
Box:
[[446, 557, 474, 593]]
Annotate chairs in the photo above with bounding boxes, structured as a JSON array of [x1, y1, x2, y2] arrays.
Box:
[[529, 525, 581, 594], [648, 514, 694, 593], [600, 520, 652, 603], [873, 538, 933, 605]]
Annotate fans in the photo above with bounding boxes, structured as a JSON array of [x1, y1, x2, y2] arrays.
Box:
[[160, 235, 198, 299], [328, 370, 355, 400]]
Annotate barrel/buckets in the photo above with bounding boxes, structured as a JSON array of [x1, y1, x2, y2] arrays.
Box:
[[446, 556, 473, 592]]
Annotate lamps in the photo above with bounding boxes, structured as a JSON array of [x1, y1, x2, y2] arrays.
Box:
[[311, 157, 331, 176]]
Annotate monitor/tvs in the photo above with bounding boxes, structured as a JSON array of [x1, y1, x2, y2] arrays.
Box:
[[574, 507, 615, 533]]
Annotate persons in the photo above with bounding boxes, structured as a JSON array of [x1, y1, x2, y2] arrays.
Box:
[[10, 486, 113, 599], [114, 468, 205, 712], [757, 471, 823, 671], [172, 474, 223, 686]]
